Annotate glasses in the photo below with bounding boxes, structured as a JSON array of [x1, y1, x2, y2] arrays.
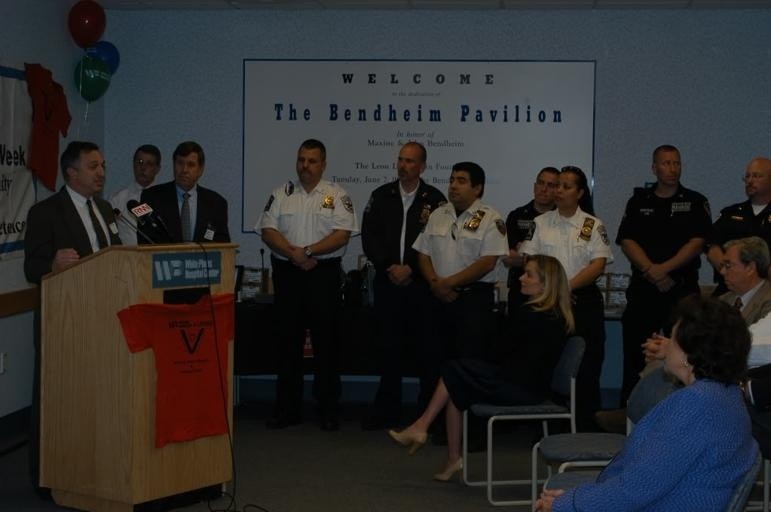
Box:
[[719, 261, 741, 268]]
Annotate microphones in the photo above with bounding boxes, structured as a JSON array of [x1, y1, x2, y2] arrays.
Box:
[[111, 208, 158, 245], [127, 199, 174, 244]]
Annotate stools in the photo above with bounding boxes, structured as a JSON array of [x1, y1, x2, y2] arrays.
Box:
[[540, 468, 605, 500]]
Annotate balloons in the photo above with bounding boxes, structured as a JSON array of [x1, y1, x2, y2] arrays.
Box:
[[83, 41, 120, 77], [68, 0, 105, 51], [74, 57, 111, 102]]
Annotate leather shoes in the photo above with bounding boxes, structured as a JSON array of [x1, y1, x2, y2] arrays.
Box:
[[266, 413, 405, 431], [432, 430, 448, 445]]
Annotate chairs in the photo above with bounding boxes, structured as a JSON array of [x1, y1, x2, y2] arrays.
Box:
[[458, 336, 587, 507], [528, 369, 681, 509], [724, 445, 766, 512]]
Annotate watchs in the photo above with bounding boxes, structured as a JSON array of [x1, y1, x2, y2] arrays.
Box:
[[304, 247, 312, 259]]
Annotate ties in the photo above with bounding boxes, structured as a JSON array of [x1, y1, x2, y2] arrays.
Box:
[[733, 298, 743, 311], [86, 201, 108, 249], [180, 195, 193, 241]]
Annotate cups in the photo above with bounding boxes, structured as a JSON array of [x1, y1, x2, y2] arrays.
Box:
[[263, 283, 273, 295], [493, 287, 500, 313]]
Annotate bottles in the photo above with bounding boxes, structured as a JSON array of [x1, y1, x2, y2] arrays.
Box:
[[302, 328, 315, 359]]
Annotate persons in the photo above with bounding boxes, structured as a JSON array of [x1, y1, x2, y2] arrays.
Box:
[[137, 142, 229, 246], [252, 139, 358, 432], [110, 144, 161, 244], [24, 141, 122, 501]]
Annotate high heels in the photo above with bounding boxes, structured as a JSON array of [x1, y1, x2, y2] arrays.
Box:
[[433, 458, 463, 482], [388, 429, 428, 454]]
